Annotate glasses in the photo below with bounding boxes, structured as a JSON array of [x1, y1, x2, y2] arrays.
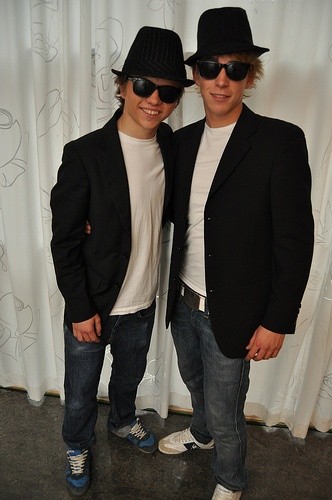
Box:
[[197, 59, 254, 81], [127, 77, 182, 103]]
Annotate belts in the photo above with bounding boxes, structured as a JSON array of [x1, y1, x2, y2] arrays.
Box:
[[177, 280, 207, 313]]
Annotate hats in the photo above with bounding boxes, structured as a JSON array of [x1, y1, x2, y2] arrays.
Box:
[[111, 26, 196, 87], [184, 7, 270, 66]]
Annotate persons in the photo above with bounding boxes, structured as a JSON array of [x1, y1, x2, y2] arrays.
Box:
[[50, 26, 197, 499], [86, 7, 315, 500]]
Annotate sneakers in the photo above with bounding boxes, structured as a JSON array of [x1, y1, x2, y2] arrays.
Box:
[[211, 483, 241, 500], [65, 443, 92, 497], [108, 419, 158, 454], [158, 426, 215, 455]]
